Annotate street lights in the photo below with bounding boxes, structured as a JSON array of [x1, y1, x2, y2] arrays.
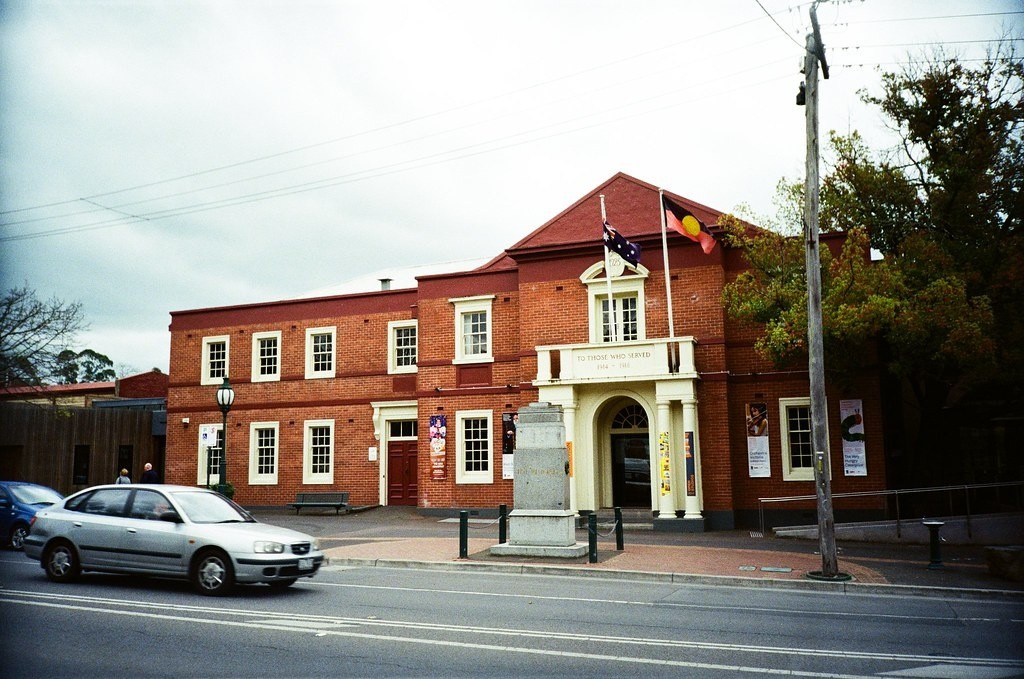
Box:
[[215, 373, 235, 497]]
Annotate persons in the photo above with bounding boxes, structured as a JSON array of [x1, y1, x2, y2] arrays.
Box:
[[140, 462, 159, 484], [429, 418, 446, 452], [505, 414, 518, 455], [746, 404, 769, 437], [116, 469, 131, 484], [145, 496, 170, 520]]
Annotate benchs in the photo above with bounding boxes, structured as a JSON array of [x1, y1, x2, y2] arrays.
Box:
[[287, 492, 349, 516]]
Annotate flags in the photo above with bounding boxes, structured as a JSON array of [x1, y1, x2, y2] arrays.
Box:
[[601, 201, 641, 268], [661, 194, 717, 255]]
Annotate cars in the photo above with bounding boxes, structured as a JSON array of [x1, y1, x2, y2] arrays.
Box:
[[23, 484, 325, 597], [0, 481, 67, 552]]
